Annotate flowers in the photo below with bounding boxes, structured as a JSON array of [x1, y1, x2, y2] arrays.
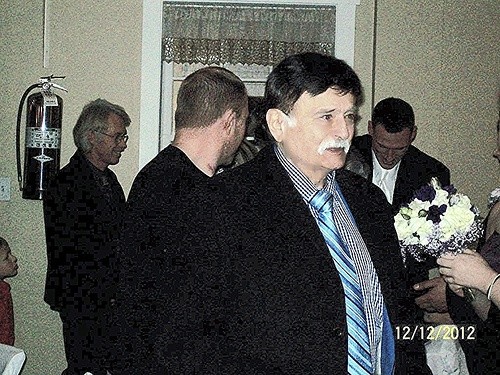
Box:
[[486, 187, 500, 211], [394, 175, 485, 304]]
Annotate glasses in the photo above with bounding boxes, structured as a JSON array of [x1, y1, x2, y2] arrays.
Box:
[[94, 130, 128, 144]]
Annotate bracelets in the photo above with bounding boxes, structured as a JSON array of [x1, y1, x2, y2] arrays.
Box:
[[487, 273, 500, 300]]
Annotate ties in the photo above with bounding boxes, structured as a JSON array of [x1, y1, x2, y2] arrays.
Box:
[[311, 191, 373, 375]]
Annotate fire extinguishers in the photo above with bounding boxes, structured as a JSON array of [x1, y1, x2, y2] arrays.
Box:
[[17, 76, 68, 200]]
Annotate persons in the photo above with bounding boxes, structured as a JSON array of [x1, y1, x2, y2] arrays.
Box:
[[415, 120, 500, 375], [150, 53, 435, 375], [0, 236, 18, 347], [343, 96, 451, 301], [106, 66, 250, 375], [43, 98, 131, 375]]
